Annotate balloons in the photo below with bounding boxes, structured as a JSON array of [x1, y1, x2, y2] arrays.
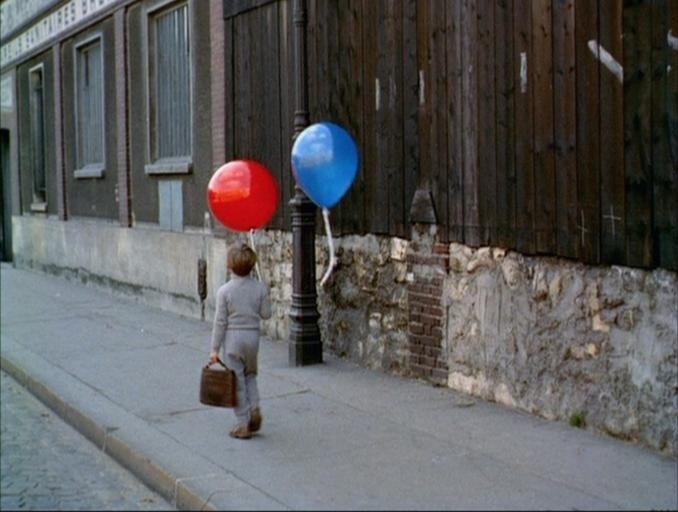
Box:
[[206, 159, 281, 233], [291, 120, 360, 210]]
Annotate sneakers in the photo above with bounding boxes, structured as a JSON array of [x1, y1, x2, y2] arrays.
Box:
[[228, 409, 263, 440]]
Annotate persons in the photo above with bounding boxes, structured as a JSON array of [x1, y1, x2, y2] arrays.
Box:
[[209, 244, 273, 439]]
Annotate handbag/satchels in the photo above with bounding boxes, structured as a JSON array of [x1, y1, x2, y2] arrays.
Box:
[[199, 358, 239, 409]]
